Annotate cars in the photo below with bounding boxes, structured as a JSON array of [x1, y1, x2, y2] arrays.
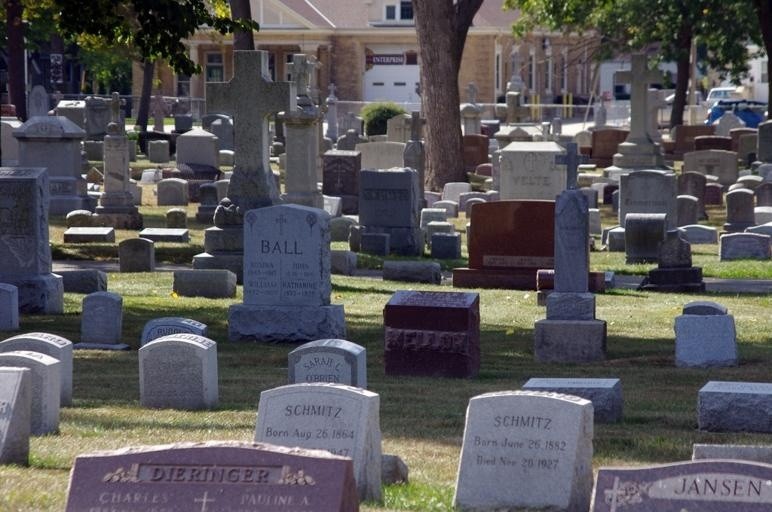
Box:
[[706, 87, 737, 103]]
